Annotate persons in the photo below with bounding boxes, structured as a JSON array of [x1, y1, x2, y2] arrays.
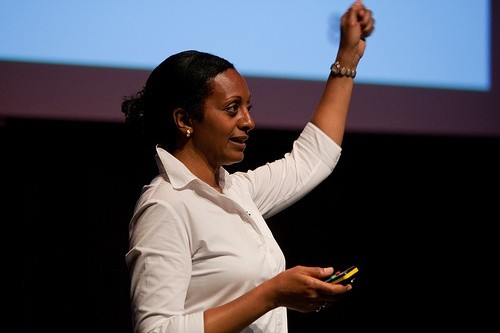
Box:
[[119, 0, 376, 333]]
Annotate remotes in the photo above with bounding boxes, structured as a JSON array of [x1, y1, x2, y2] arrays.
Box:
[[327, 267, 360, 285]]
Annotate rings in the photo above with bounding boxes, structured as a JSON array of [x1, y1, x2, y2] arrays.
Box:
[[316, 303, 327, 312]]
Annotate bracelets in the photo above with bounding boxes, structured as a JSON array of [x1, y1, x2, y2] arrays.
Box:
[[330, 62, 356, 78]]
